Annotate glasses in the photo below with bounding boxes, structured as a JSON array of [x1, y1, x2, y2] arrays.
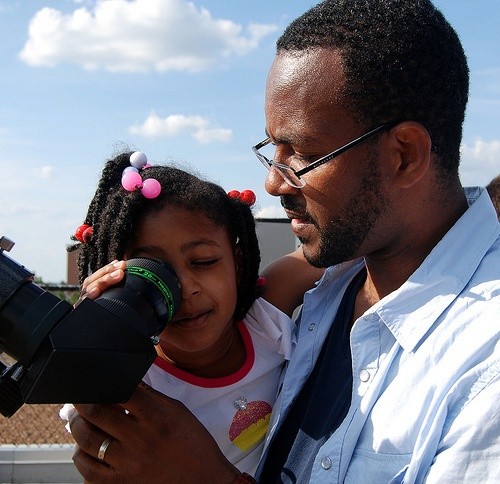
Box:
[[253, 120, 438, 188]]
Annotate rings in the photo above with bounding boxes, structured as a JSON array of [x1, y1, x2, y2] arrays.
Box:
[[98, 438, 112, 463]]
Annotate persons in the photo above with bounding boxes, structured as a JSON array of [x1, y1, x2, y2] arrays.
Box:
[[69, 1, 499, 484], [59, 152, 500, 476]]
